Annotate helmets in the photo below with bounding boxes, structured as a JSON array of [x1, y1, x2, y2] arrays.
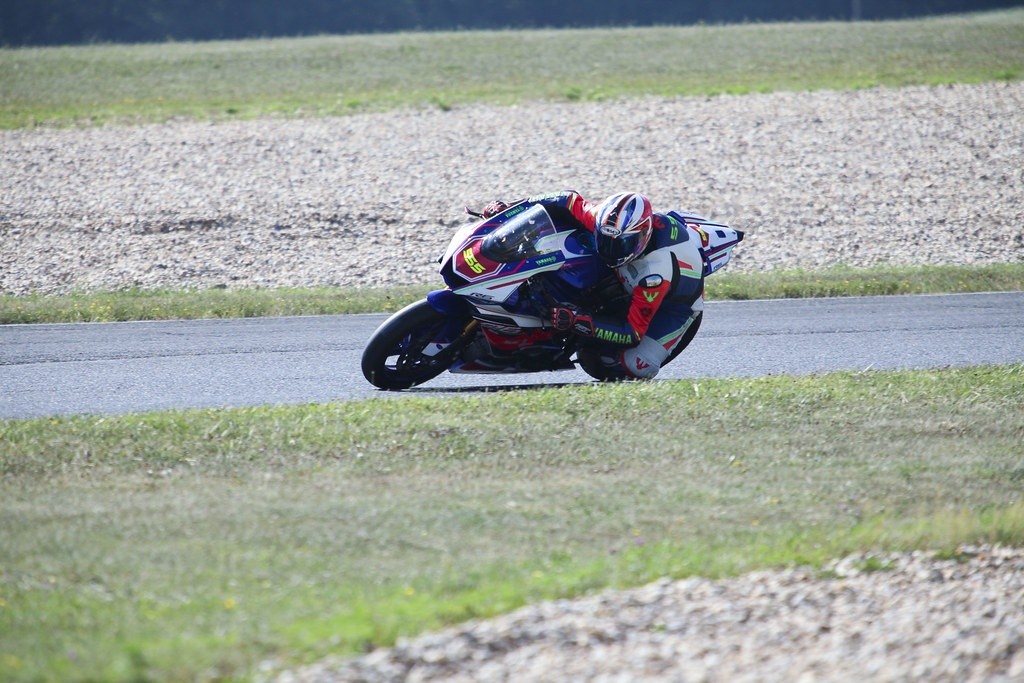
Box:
[[594, 194, 653, 267]]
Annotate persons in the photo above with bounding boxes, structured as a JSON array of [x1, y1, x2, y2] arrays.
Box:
[[480, 189, 703, 380]]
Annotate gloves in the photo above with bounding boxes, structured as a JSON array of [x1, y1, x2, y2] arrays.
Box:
[[484, 201, 512, 218], [554, 300, 594, 337]]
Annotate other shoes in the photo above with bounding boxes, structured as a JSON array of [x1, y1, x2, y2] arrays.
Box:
[[599, 349, 621, 367]]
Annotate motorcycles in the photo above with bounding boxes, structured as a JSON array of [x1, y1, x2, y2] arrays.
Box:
[[361, 197, 745, 389]]
